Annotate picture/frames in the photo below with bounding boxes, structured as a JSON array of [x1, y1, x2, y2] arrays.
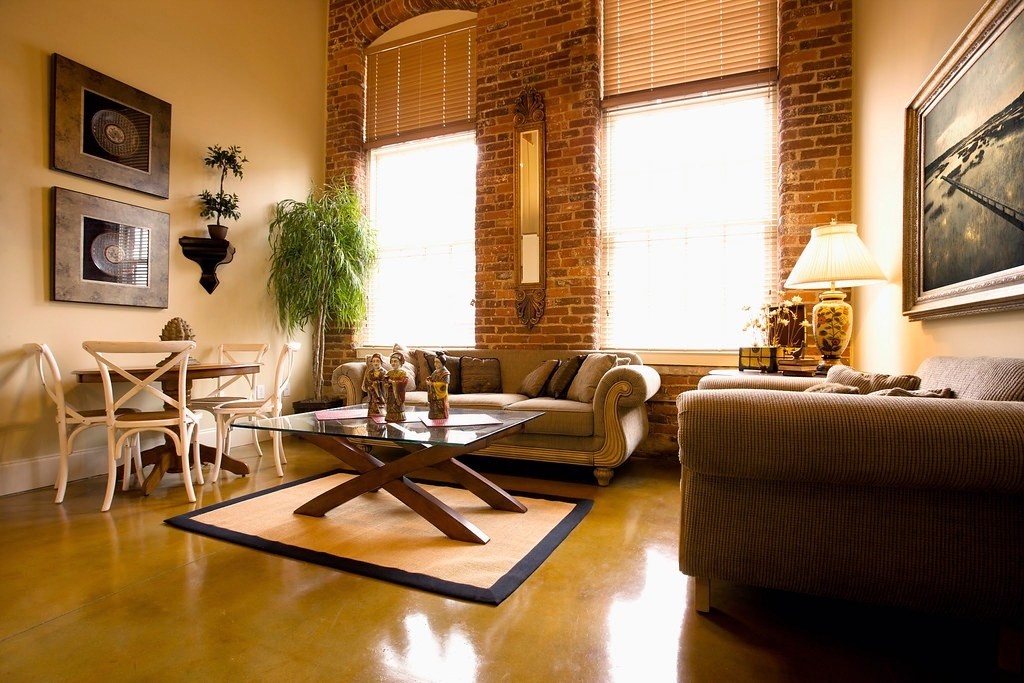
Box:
[[902, 0, 1024, 321]]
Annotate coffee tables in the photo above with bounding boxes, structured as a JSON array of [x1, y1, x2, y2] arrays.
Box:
[[231, 403, 546, 545]]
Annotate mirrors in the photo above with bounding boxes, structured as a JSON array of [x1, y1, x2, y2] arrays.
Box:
[[512, 86, 546, 330]]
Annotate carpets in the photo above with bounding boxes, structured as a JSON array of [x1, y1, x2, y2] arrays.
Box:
[[165, 467, 596, 606]]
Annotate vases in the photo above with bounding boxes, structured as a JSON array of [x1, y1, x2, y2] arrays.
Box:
[[765, 347, 779, 372]]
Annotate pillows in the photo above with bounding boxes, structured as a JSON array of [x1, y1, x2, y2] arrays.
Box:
[[365, 355, 417, 391], [617, 357, 631, 366], [392, 343, 421, 391], [803, 382, 860, 394], [516, 359, 562, 398], [825, 365, 922, 396], [866, 388, 952, 398], [424, 354, 461, 394], [561, 353, 618, 402], [541, 354, 588, 400], [458, 355, 502, 394], [414, 350, 450, 391]]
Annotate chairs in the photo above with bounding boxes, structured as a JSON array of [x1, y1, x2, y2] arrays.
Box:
[[188, 344, 270, 466], [22, 343, 146, 503], [211, 341, 301, 482], [83, 341, 205, 511]]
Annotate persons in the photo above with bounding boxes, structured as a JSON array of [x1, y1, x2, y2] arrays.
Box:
[[427, 351, 450, 418], [382, 352, 409, 420], [364, 354, 386, 415]]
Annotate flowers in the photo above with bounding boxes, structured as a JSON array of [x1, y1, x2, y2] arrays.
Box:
[[741, 292, 814, 346]]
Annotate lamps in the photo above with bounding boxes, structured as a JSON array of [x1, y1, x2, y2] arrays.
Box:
[[783, 220, 887, 374]]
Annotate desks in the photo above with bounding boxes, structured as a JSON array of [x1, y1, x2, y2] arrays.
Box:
[[71, 364, 265, 501], [708, 367, 785, 377]]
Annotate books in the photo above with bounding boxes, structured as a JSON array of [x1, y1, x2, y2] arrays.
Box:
[[778, 359, 819, 377]]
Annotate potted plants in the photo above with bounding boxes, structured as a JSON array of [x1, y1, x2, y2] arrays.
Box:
[[267, 173, 380, 415], [197, 143, 249, 238]]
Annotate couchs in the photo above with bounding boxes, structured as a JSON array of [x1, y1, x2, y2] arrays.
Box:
[[675, 356, 1024, 615], [333, 348, 661, 486]]
